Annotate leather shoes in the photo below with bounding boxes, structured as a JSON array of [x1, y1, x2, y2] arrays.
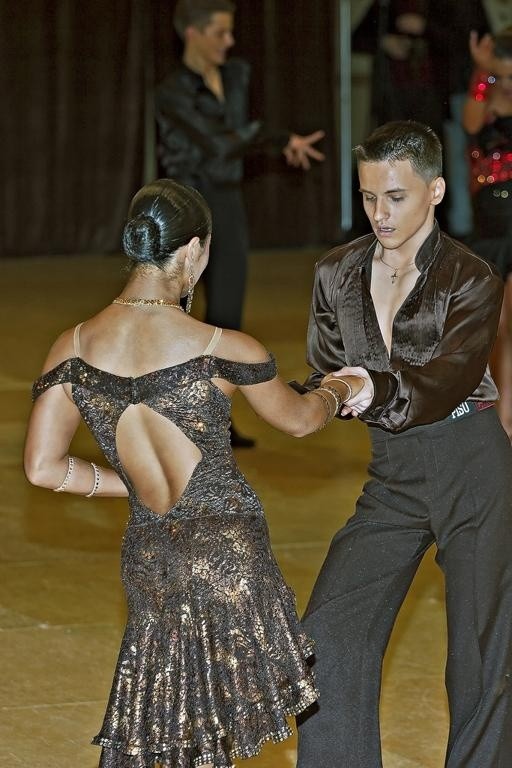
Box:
[[231, 425, 256, 449]]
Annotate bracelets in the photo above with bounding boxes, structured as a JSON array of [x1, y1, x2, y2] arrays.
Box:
[[54, 454, 74, 492], [469, 72, 491, 102], [314, 385, 342, 421], [308, 391, 331, 430], [326, 377, 351, 403], [86, 460, 101, 499]]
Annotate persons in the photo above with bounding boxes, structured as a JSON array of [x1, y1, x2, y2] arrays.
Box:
[[155, 0, 325, 447], [21, 178, 365, 768], [462, 29, 511, 438], [287, 120, 512, 768], [352, 2, 493, 146]]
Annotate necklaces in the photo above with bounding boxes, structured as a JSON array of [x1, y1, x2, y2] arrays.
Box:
[[111, 296, 186, 313]]
